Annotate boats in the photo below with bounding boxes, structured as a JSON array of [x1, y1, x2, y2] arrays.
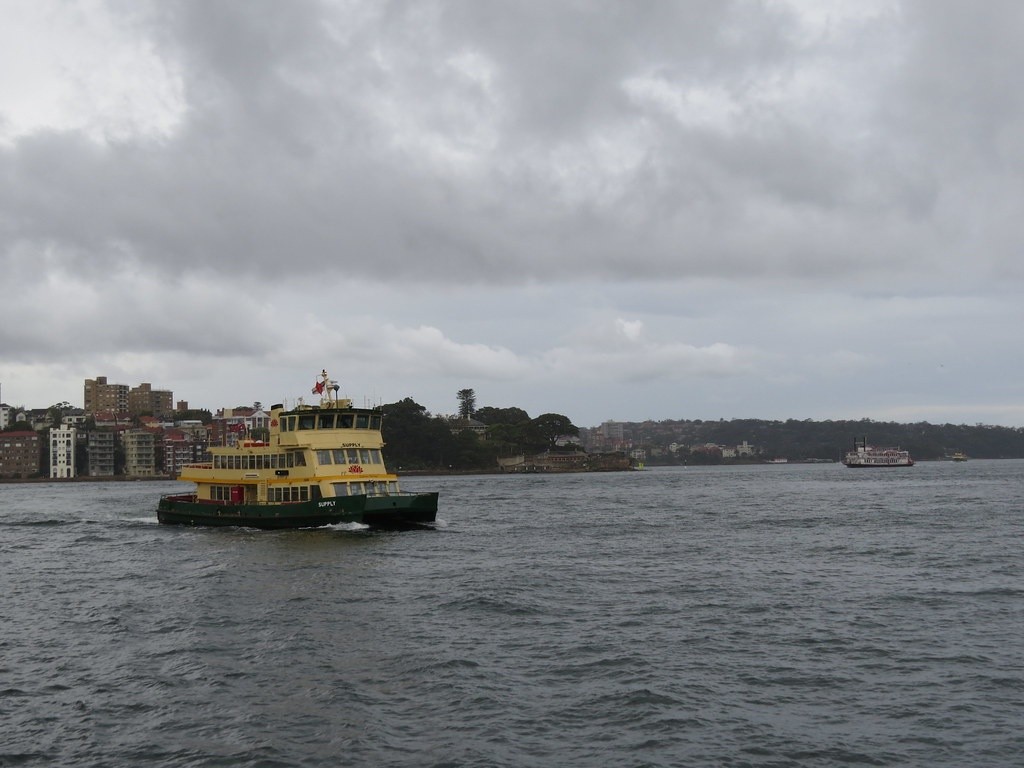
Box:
[[953, 453, 968, 461], [842, 437, 914, 468], [157, 369, 439, 530]]
